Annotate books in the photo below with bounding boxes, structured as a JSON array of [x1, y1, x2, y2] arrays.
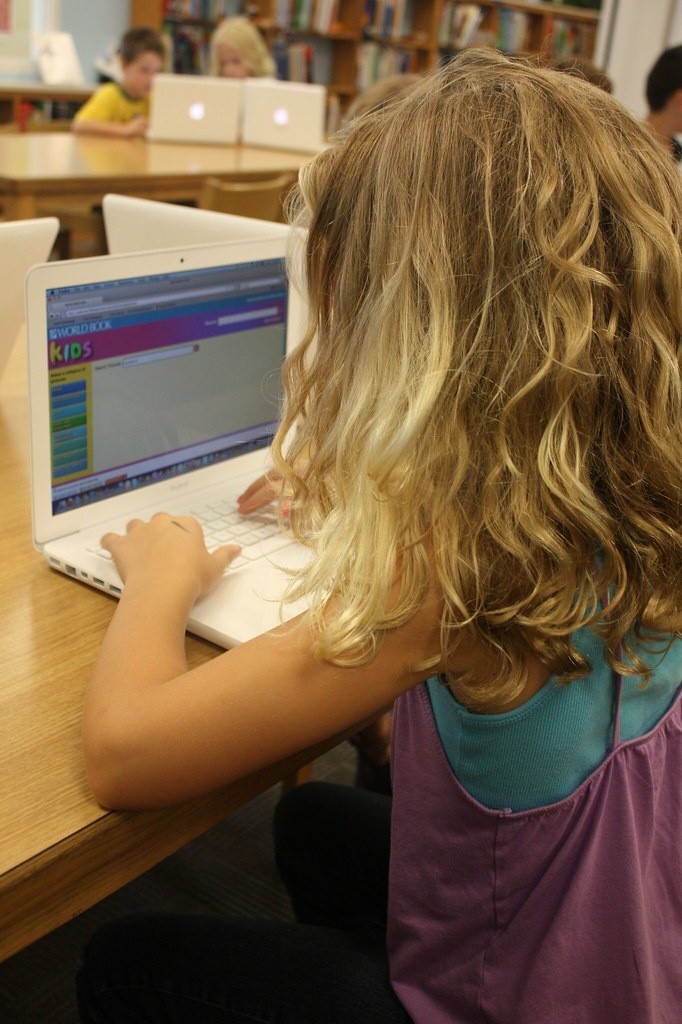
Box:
[[160, 0, 599, 114]]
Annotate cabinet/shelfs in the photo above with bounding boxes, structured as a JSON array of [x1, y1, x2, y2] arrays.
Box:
[[128, 0, 603, 117]]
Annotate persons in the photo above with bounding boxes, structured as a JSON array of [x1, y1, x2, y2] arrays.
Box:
[[208, 15, 277, 79], [78, 45, 682, 1024], [71, 29, 166, 138], [640, 44, 682, 169]]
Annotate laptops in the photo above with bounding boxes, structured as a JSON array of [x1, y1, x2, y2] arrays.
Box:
[[141, 75, 243, 146], [243, 79, 335, 154], [0, 193, 342, 651]]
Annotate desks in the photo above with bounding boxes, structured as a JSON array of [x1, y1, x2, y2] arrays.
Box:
[[0, 128, 316, 258], [0, 81, 94, 124], [0, 326, 393, 967]]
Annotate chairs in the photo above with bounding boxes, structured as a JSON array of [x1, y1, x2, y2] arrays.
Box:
[[199, 172, 295, 221]]
